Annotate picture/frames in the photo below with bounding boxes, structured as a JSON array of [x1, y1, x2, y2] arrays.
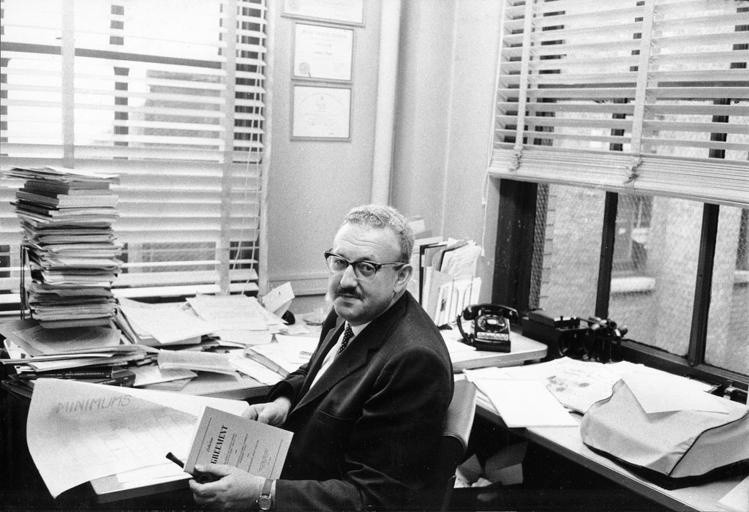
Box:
[[288, 82, 353, 144], [290, 20, 356, 85], [279, 0, 370, 29]]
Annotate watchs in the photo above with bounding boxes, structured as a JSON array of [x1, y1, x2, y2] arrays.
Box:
[[257, 478, 273, 510]]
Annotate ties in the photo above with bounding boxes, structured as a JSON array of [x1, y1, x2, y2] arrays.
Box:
[[334, 321, 351, 357]]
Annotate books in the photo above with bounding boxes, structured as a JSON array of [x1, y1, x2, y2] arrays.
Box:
[[181, 407, 295, 496], [9, 165, 121, 326], [228, 217, 487, 384]]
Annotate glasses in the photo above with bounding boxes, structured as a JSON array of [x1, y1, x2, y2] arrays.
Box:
[[324, 248, 402, 282]]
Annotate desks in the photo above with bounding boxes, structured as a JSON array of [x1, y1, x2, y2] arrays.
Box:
[[452, 354, 749, 512], [4, 320, 547, 504]]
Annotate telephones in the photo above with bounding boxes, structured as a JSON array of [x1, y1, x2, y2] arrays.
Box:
[[463, 302, 518, 352]]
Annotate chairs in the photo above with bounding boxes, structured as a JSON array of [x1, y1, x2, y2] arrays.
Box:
[[417, 379, 484, 512]]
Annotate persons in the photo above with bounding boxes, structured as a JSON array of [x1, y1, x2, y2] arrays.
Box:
[[189, 205, 454, 511]]
